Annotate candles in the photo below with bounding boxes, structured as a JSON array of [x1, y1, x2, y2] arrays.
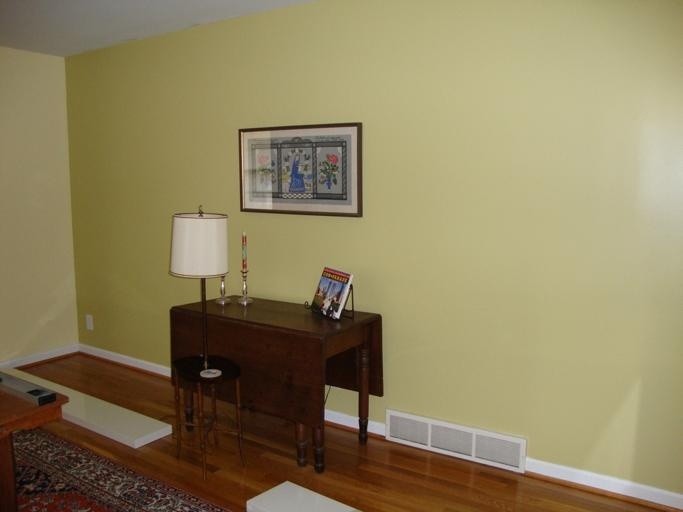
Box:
[[241, 233, 247, 271]]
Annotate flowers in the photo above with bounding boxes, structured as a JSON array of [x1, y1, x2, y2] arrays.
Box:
[[319, 154, 338, 191], [255, 154, 276, 183]]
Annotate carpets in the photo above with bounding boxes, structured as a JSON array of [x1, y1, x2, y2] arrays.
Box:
[[9, 427, 229, 512]]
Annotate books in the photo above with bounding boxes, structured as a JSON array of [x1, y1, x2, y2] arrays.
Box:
[[309, 266, 353, 320]]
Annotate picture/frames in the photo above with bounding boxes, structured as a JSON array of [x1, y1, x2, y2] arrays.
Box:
[[238, 123, 362, 218]]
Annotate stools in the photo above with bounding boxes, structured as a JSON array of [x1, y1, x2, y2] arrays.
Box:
[[171, 354, 247, 481]]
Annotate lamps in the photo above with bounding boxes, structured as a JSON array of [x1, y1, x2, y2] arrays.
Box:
[[167, 205, 244, 380]]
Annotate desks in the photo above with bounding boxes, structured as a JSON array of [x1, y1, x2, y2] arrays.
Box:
[[166, 295, 383, 474], [1, 372, 68, 512]]
